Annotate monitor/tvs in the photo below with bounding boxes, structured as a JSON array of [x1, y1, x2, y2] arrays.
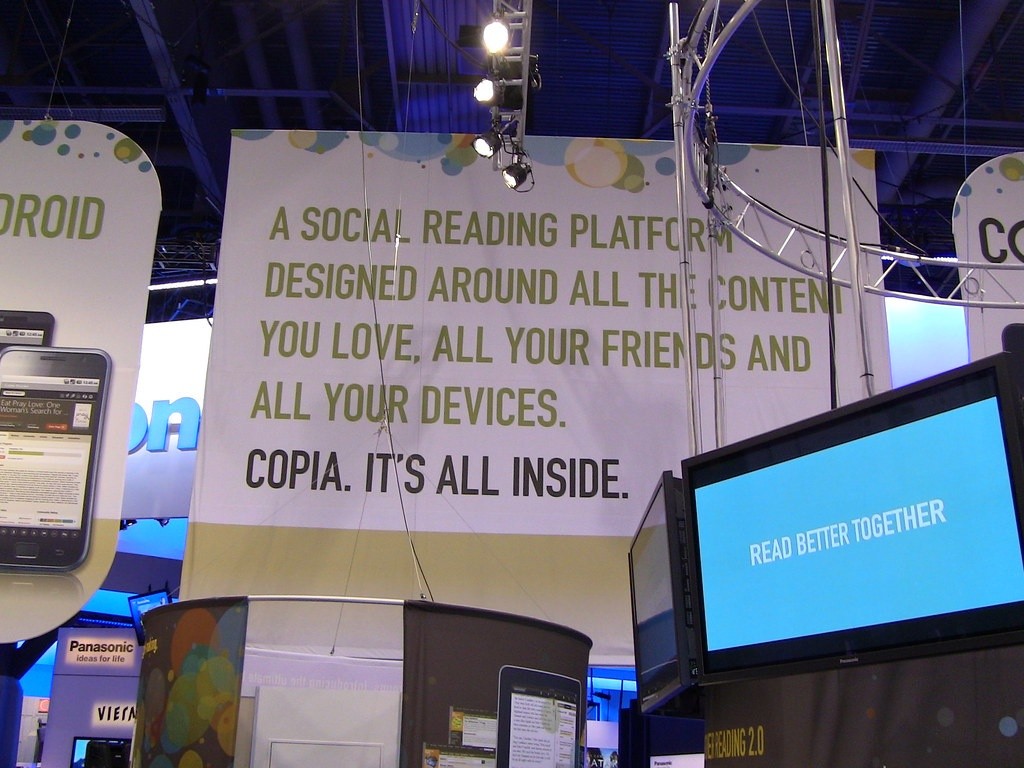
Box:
[[127, 588, 172, 647], [70, 736, 132, 768], [685, 365, 1024, 685], [629, 470, 700, 715], [497, 665, 581, 768]]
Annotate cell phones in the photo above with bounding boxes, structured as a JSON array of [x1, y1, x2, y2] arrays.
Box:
[[0, 346, 112, 575], [0, 310, 55, 352]]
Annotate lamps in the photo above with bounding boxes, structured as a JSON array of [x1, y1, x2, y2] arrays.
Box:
[[482, 17, 511, 50], [473, 79, 505, 106], [502, 161, 532, 189], [469, 127, 503, 159]]
